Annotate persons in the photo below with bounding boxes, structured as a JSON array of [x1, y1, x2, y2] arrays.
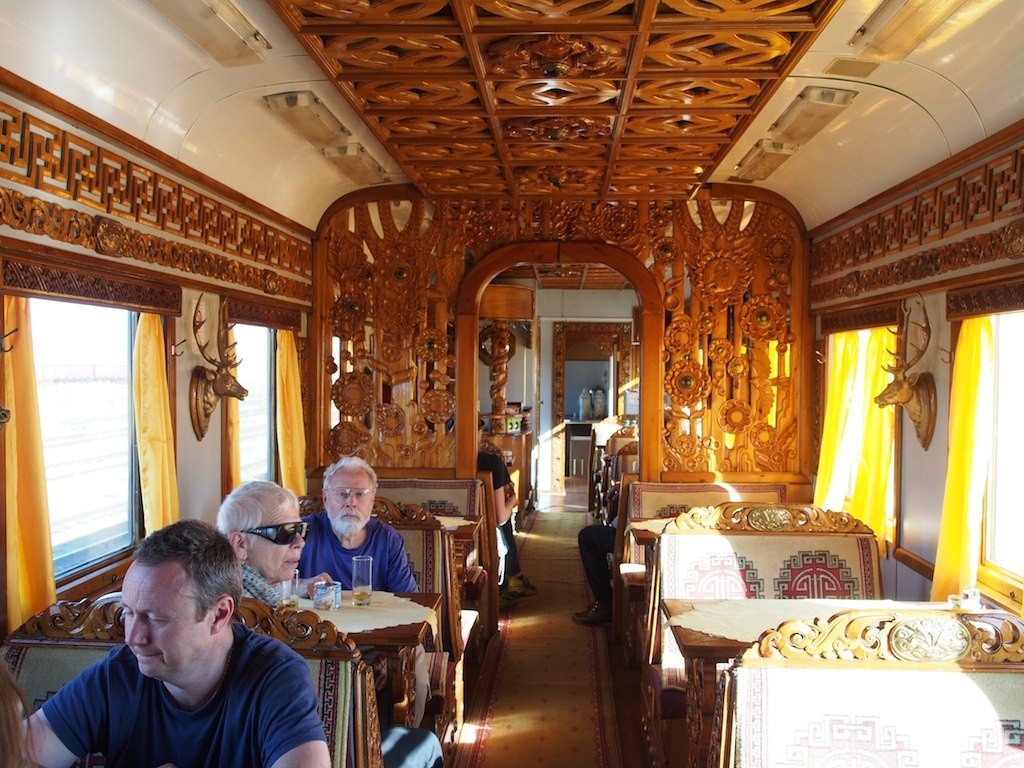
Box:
[[217, 480, 309, 620], [571, 514, 618, 627], [297, 456, 421, 667], [14, 520, 333, 768], [477, 419, 538, 610]]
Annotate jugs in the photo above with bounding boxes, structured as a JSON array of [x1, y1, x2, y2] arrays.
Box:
[[579, 387, 592, 421], [593, 390, 606, 419]]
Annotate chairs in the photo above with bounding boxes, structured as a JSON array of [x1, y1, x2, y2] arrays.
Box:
[[641, 502, 889, 768], [584, 416, 639, 530], [618, 477, 791, 668], [5, 593, 360, 768], [710, 611, 1024, 768]]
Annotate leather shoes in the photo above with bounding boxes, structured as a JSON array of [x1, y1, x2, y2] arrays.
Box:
[[571, 601, 613, 625]]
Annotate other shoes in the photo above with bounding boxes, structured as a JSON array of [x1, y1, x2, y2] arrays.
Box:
[[508, 574, 538, 597], [499, 590, 519, 610]]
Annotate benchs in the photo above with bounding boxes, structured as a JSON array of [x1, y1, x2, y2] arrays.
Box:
[[372, 501, 461, 750], [367, 475, 494, 648]]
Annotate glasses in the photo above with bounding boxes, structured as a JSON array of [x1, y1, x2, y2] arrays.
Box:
[[241, 521, 310, 545], [322, 487, 376, 503]]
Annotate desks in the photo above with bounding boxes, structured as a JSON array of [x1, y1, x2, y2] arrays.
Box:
[[662, 598, 1001, 768], [387, 506, 486, 599], [628, 518, 824, 660], [277, 588, 447, 735]]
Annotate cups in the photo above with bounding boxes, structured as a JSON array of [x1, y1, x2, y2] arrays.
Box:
[[313, 581, 340, 613], [353, 556, 372, 607], [283, 570, 298, 614]]
